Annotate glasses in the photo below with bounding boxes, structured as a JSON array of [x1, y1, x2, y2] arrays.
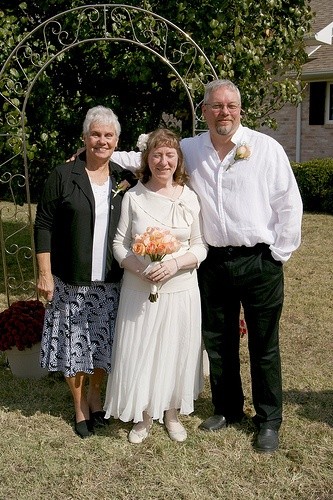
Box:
[[205, 103, 240, 110]]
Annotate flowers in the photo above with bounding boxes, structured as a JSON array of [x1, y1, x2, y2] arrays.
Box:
[[238, 319, 248, 337], [226, 145, 250, 171], [0, 299, 46, 351], [136, 133, 149, 151], [130, 226, 178, 303], [112, 175, 131, 199]]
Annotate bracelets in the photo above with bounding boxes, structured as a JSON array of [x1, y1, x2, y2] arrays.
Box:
[[173, 258, 178, 272]]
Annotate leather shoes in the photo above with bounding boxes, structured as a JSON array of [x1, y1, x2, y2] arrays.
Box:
[[254, 426, 279, 451], [198, 414, 226, 431]]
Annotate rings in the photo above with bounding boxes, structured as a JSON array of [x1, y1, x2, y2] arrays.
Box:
[[163, 271, 166, 276], [160, 263, 162, 268]]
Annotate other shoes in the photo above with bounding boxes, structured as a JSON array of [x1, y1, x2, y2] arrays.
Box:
[[128, 417, 153, 444], [75, 407, 91, 436], [163, 416, 188, 442], [88, 407, 110, 427]]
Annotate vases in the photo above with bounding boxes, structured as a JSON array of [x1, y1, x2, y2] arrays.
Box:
[[3, 342, 49, 379]]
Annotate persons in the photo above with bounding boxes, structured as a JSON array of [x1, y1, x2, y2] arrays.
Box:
[[66, 79, 304, 454], [33, 105, 139, 439], [103, 129, 209, 444]]
